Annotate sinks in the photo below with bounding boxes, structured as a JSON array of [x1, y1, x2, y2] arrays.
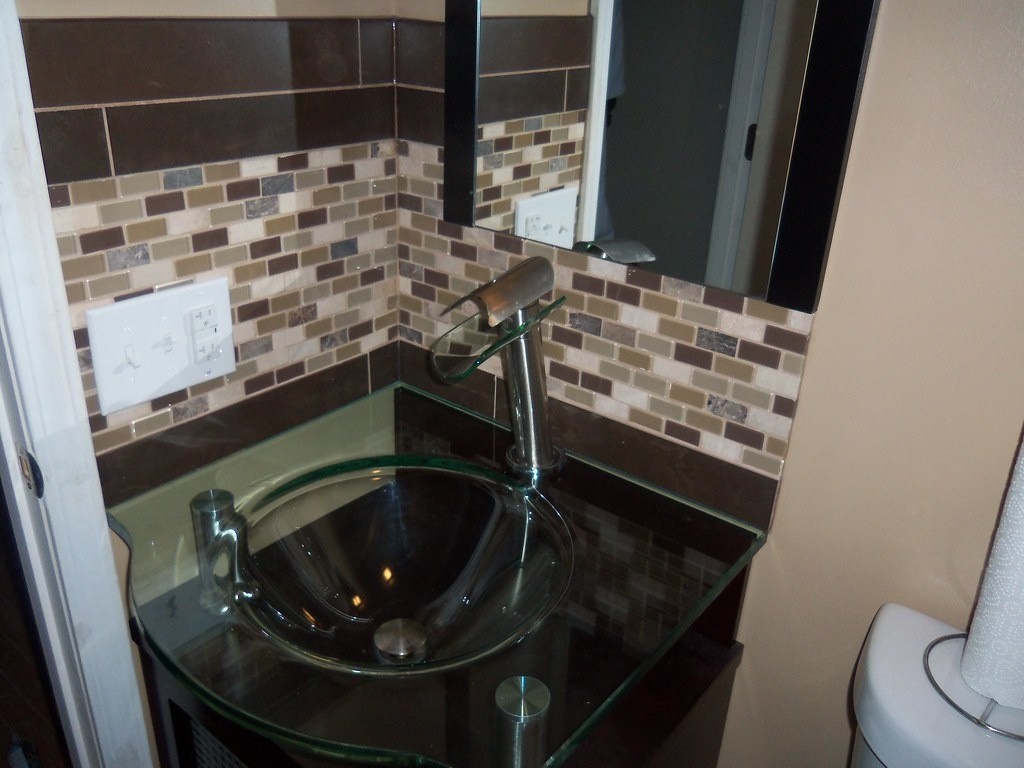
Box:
[[212, 453, 584, 677]]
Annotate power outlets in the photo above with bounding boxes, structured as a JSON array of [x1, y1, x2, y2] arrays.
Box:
[[514, 188, 577, 251], [87, 275, 237, 415]]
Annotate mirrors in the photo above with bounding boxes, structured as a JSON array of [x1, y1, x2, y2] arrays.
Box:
[[443, 0, 881, 314]]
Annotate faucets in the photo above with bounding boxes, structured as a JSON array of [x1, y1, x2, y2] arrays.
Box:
[[424, 256, 567, 480]]
